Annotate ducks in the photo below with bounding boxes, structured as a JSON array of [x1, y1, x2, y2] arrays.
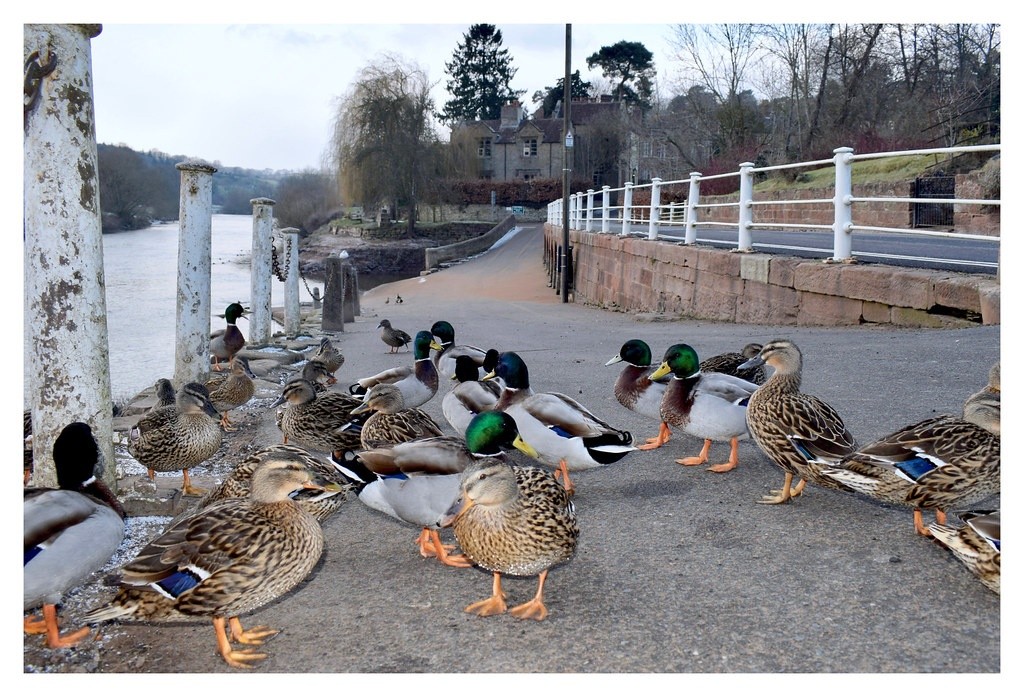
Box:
[[820, 361, 1000, 595], [604, 339, 768, 473], [736, 337, 861, 504], [23, 304, 642, 673], [385, 293, 403, 304]]
